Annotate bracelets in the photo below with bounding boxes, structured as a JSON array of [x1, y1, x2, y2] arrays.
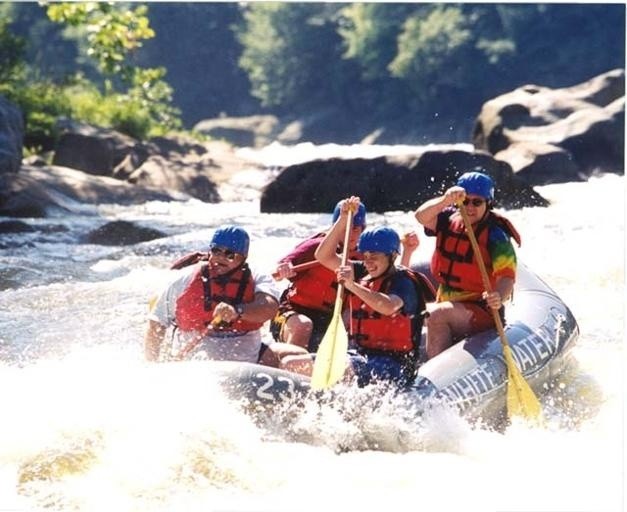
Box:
[[235, 303, 244, 320]]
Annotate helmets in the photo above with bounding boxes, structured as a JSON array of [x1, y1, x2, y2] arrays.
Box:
[[356, 226, 401, 256], [332, 198, 366, 225], [210, 225, 249, 257], [456, 172, 496, 200]]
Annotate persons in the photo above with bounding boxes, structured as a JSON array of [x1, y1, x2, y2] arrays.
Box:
[[413, 168, 519, 358], [275, 195, 422, 389], [142, 224, 283, 366], [270, 198, 420, 346]]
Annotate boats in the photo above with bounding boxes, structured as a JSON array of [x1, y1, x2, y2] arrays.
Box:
[[133, 256, 578, 454]]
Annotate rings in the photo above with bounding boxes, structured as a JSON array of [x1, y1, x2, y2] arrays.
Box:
[[224, 313, 227, 318]]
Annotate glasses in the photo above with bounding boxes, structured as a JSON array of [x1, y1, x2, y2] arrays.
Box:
[[211, 247, 240, 261], [461, 198, 488, 207]]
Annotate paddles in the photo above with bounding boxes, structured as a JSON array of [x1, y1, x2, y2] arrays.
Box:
[[456, 197, 543, 422], [311, 203, 356, 392]]
